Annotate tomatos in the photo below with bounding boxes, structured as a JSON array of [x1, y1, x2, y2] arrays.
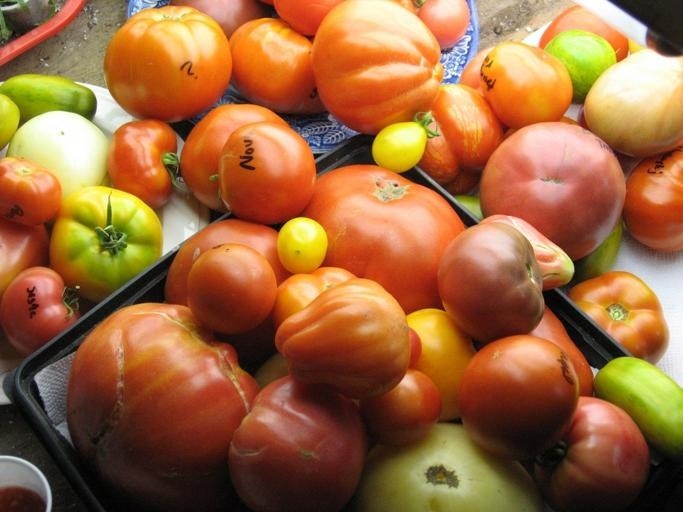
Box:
[[0, 1, 682, 510]]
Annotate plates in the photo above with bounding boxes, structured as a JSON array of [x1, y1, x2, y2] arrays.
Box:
[[130, 1, 481, 166], [0, 78, 211, 402], [1, 1, 85, 69]]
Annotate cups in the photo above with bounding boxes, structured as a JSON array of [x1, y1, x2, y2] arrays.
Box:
[[0, 451, 57, 509]]
